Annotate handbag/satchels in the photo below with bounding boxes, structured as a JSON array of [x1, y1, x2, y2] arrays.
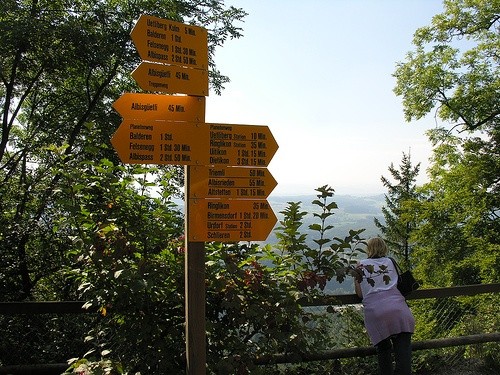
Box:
[[388, 257, 419, 296]]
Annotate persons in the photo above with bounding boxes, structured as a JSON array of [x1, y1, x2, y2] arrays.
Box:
[[355, 238, 415, 374]]
